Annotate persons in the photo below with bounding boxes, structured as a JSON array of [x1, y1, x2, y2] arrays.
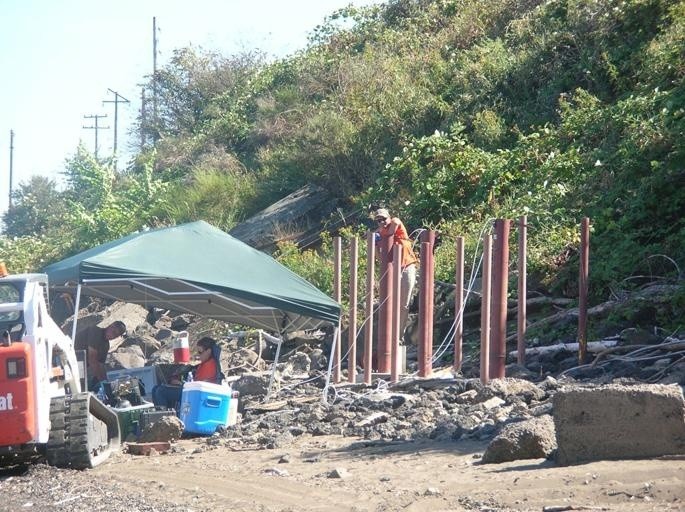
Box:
[[152, 337, 218, 412], [53, 321, 125, 400], [376, 208, 416, 344]]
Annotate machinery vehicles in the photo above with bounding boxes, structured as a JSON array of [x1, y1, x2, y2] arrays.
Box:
[[0, 272, 122, 476]]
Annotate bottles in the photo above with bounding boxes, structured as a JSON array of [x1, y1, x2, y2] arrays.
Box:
[[3, 330, 11, 348], [188, 372, 193, 383]]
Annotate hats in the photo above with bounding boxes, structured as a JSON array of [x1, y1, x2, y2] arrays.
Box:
[[376, 209, 389, 218]]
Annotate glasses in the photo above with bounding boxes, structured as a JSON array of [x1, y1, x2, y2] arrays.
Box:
[[378, 217, 386, 222]]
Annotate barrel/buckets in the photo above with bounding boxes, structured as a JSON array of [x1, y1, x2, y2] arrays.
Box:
[[172, 331, 190, 362]]
